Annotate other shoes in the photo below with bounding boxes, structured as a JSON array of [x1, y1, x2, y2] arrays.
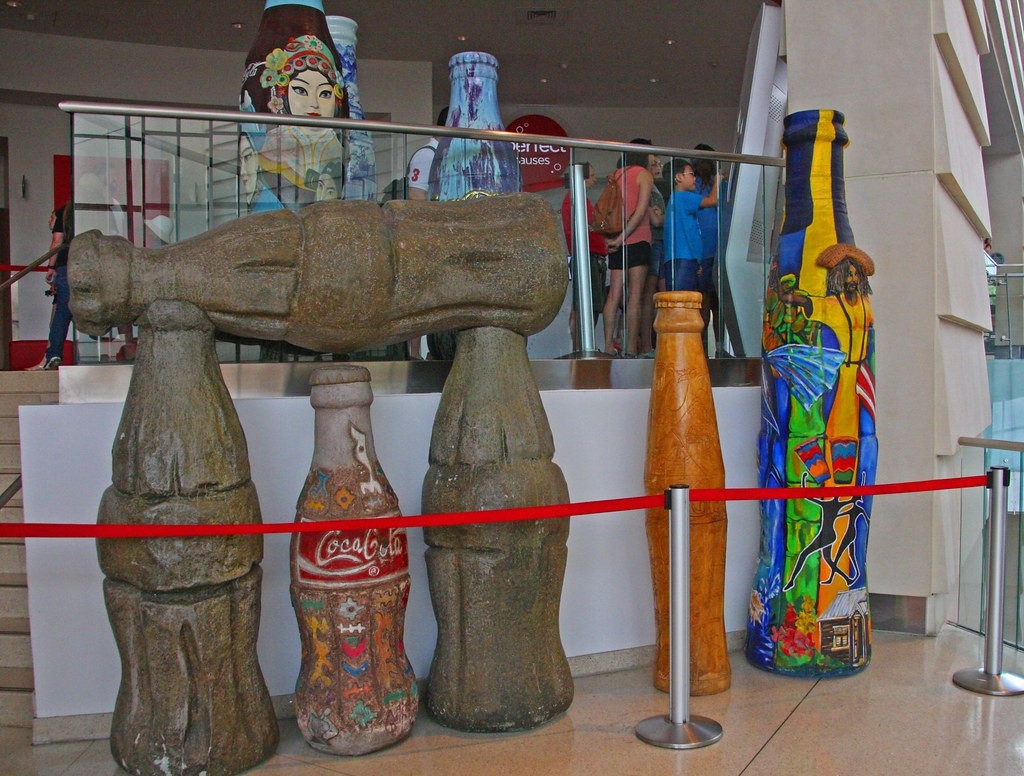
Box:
[[625, 353, 641, 359], [641, 349, 656, 357], [44, 357, 61, 369], [612, 349, 622, 358], [715, 350, 735, 358]]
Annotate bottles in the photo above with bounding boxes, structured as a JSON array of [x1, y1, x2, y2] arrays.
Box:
[[94, 297, 281, 776], [289, 365, 419, 755], [422, 326, 575, 734], [322, 15, 381, 203], [238, 0, 350, 214], [68, 192, 570, 356], [743, 108, 873, 679], [427, 52, 520, 203], [643, 291, 732, 697]]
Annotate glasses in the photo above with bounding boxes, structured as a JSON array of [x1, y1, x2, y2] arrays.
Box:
[[675, 172, 694, 180]]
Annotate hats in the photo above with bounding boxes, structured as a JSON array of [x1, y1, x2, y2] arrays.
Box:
[[144, 215, 173, 243]]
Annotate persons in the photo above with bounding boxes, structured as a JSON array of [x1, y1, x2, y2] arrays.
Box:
[[407, 107, 452, 365], [26, 165, 175, 370], [561, 138, 735, 358]]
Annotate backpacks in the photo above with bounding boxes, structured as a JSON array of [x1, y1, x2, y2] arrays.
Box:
[[381, 145, 437, 205], [591, 165, 633, 234]]
